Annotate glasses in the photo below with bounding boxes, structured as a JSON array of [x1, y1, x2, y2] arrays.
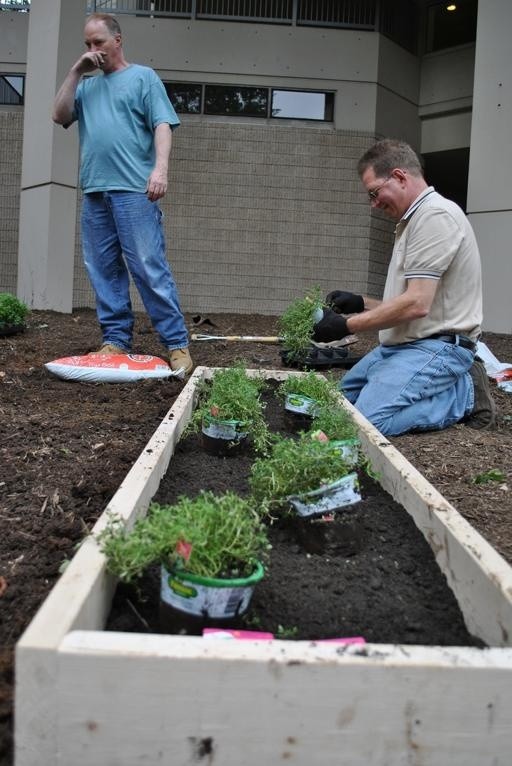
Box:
[[367, 172, 407, 200]]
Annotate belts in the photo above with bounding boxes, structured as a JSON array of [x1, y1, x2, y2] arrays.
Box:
[[429, 334, 475, 351]]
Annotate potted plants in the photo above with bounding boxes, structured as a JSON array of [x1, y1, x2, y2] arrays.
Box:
[[180, 354, 281, 455], [276, 365, 362, 438], [95, 489, 272, 620], [273, 283, 324, 361], [249, 430, 360, 519]]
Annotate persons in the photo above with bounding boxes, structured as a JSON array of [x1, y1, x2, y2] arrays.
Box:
[[51, 14, 194, 376], [310, 140, 496, 438]]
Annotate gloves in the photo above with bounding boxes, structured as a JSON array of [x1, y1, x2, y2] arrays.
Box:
[[326, 290, 364, 314], [311, 306, 355, 343]]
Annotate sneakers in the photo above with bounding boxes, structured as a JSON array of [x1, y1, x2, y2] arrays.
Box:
[[168, 347, 194, 375], [88, 344, 128, 355], [466, 361, 495, 430]]
